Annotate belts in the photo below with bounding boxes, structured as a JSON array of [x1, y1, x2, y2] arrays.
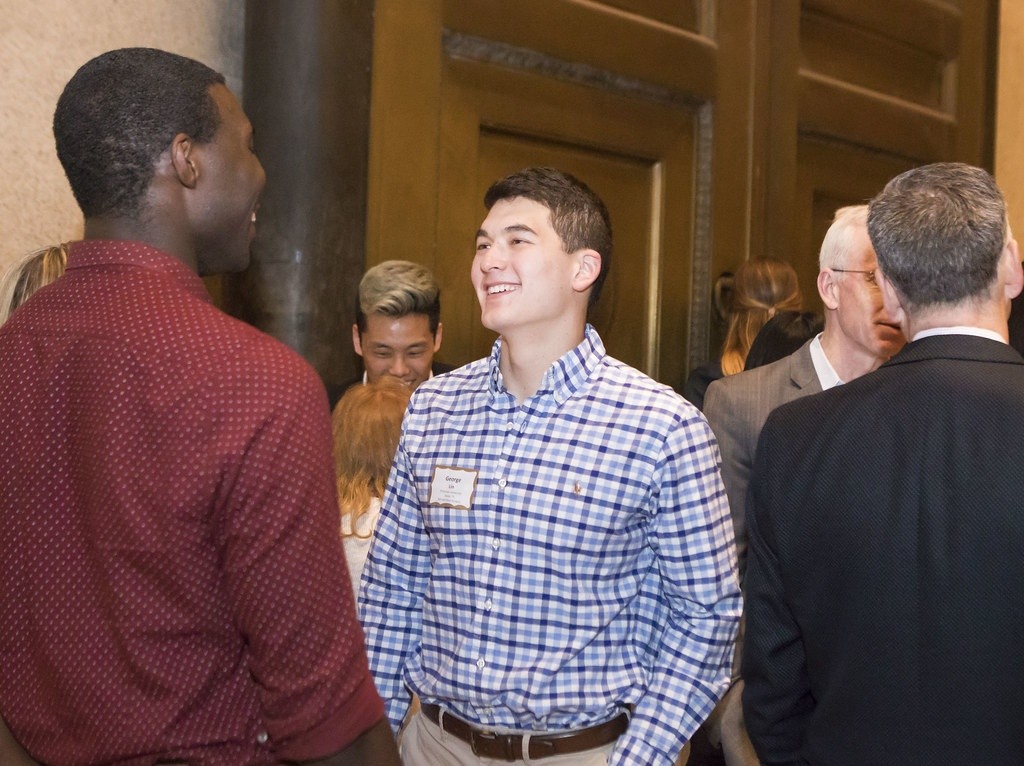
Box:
[[420, 700, 628, 762]]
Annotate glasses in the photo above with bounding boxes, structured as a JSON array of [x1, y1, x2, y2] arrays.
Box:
[[832, 269, 878, 286]]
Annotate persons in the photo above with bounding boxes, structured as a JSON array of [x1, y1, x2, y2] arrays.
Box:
[[329, 379, 412, 600], [684, 251, 824, 414], [327, 259, 459, 416], [0, 47, 403, 766], [742, 162, 1024, 766], [357, 165, 744, 766], [701, 204, 907, 766]]
